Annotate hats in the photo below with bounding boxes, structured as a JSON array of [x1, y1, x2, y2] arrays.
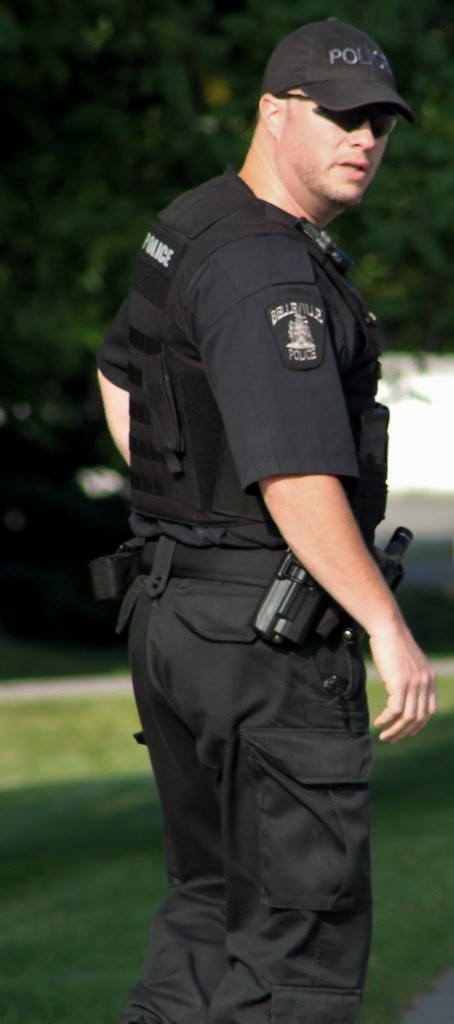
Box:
[[261, 18, 418, 124]]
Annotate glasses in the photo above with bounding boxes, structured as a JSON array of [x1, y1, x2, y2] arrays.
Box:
[[274, 93, 398, 139]]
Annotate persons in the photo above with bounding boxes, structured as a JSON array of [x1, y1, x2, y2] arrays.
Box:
[[89, 17, 434, 1024]]
